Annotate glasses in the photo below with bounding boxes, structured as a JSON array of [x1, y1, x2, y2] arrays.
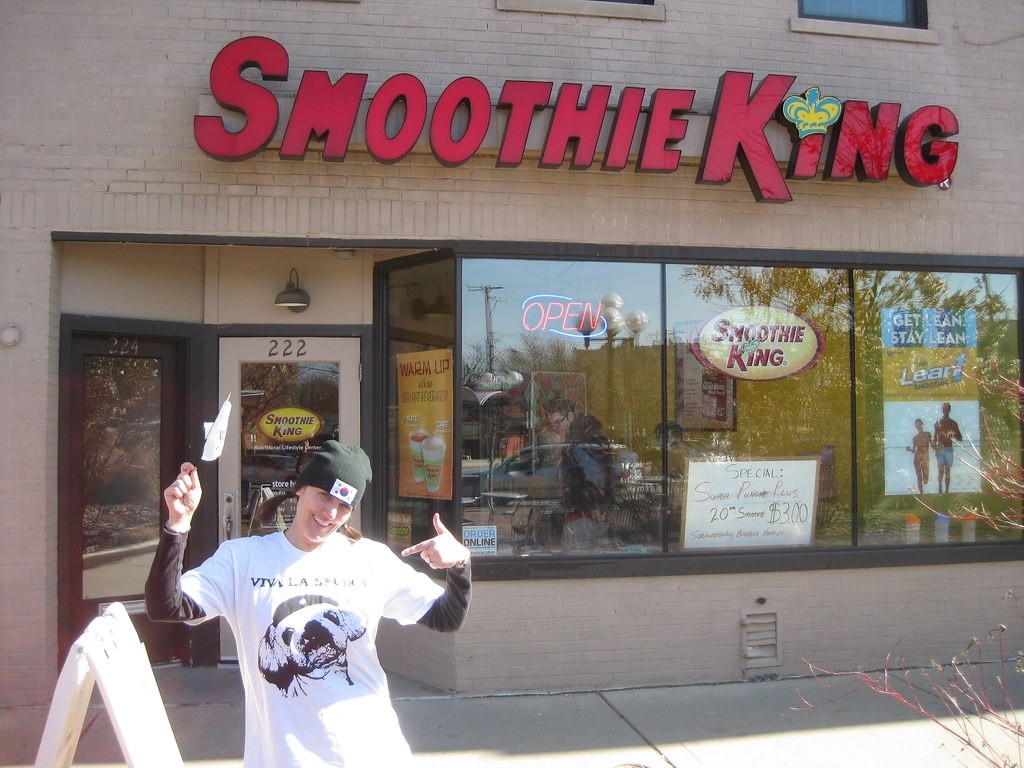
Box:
[[547, 413, 568, 430]]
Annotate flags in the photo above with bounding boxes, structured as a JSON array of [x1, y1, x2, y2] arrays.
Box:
[[200, 401, 231, 461]]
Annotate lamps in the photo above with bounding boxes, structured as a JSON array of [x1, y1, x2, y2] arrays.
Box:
[[273, 268, 310, 313], [408, 279, 452, 321]]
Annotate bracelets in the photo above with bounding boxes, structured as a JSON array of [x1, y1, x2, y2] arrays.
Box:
[[455, 548, 469, 568]]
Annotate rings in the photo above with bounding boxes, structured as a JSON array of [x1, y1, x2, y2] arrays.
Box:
[[428, 556, 431, 563]]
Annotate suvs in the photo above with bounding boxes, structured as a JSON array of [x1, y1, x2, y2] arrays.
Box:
[[461, 443, 644, 506]]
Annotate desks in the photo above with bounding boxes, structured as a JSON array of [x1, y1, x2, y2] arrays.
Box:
[[481, 491, 528, 545]]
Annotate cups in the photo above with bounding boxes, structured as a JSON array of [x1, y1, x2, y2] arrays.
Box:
[[407, 427, 446, 494]]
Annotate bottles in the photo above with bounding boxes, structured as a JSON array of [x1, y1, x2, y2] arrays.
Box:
[[960, 513, 977, 541], [903, 511, 921, 545], [933, 511, 950, 544]]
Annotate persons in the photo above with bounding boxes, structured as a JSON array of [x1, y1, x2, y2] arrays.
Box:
[[656, 421, 696, 505], [546, 398, 611, 547], [146, 441, 471, 768], [906, 403, 963, 494]]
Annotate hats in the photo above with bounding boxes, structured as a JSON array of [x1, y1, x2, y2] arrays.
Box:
[[296, 440, 373, 511]]
